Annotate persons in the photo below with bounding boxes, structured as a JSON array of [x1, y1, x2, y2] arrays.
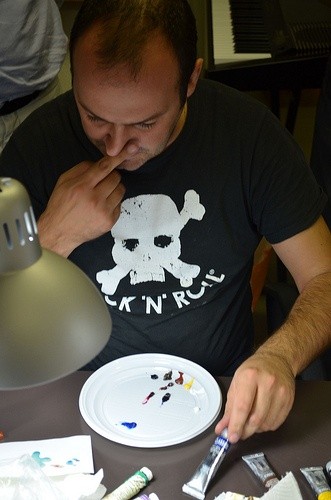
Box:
[[0, 0, 331, 443], [0, 1, 72, 150]]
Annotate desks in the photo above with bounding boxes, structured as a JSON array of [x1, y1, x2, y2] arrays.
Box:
[[1, 370, 331, 500]]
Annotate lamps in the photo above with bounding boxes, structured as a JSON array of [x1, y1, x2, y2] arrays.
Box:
[[1, 178, 113, 390]]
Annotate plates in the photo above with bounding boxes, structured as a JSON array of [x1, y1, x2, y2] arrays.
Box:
[[79, 353, 223, 447]]
